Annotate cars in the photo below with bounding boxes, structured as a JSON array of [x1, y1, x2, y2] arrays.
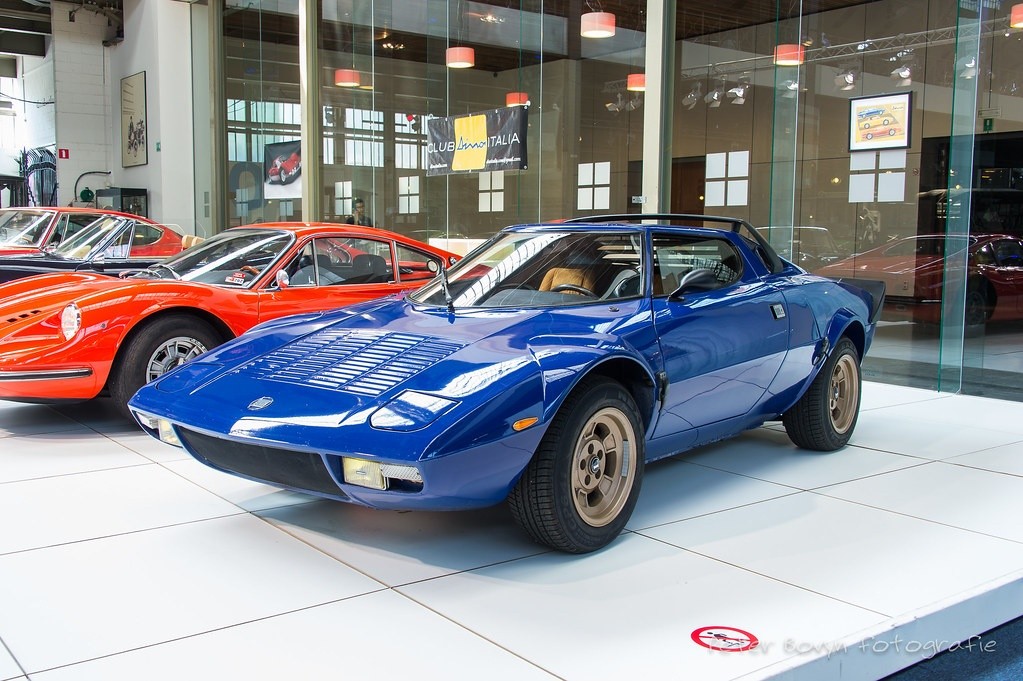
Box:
[[269, 152, 301, 185]]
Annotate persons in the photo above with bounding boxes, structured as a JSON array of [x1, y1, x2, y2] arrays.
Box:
[[36, 223, 62, 245], [346, 198, 372, 227]]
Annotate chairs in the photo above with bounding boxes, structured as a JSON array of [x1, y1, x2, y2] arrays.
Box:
[[297, 254, 332, 271], [652, 265, 680, 296], [350, 253, 384, 284], [535, 266, 595, 297]]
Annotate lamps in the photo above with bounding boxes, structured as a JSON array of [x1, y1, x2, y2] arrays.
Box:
[[334, 25, 361, 87], [506, 48, 529, 107], [444, 1, 475, 69], [1010, 1, 1023, 29], [580, 0, 616, 39], [605, 8, 975, 111]]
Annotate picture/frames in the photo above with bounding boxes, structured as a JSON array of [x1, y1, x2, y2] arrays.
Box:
[[120, 71, 148, 168], [846, 90, 913, 152]]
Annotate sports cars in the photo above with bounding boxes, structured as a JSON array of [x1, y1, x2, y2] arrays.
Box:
[[0, 205, 278, 285], [751, 223, 857, 270], [128, 211, 888, 555], [810, 233, 1023, 331], [404, 228, 496, 245], [0, 220, 493, 420]]
